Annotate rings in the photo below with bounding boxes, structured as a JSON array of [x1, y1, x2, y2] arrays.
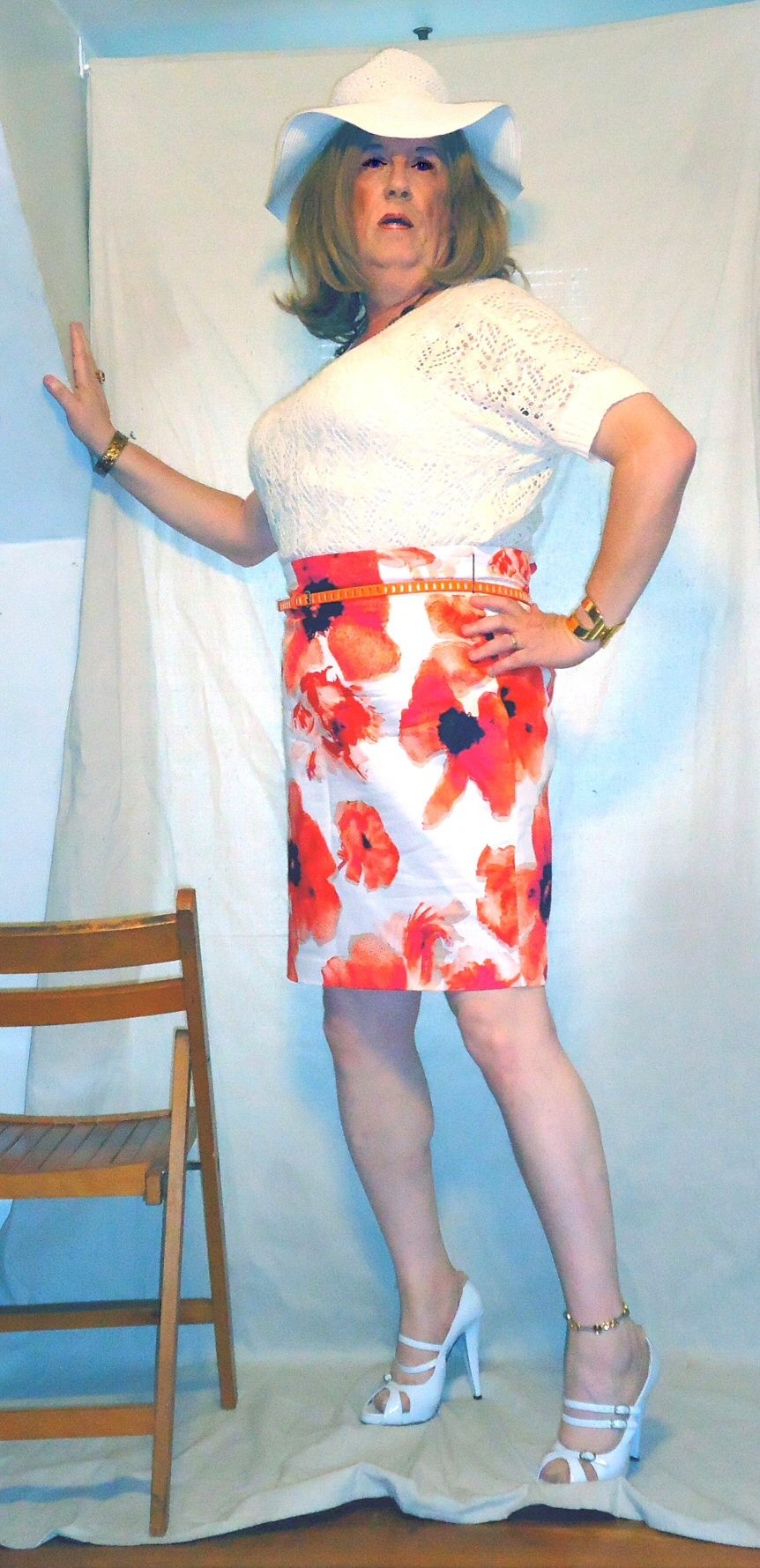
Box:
[[96, 370, 107, 384], [509, 633, 517, 650]]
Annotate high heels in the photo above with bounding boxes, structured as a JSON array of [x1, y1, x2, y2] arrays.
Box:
[[360, 1278, 483, 1426], [536, 1335, 660, 1484]]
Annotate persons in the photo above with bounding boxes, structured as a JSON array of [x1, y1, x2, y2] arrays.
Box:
[[46, 46, 700, 1487]]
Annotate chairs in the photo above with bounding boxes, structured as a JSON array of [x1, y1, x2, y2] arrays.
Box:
[[3, 887, 242, 1536]]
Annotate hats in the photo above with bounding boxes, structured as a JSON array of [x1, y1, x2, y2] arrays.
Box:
[[264, 48, 524, 225]]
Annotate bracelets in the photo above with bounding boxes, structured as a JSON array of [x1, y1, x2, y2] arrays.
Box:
[[566, 596, 628, 649], [92, 432, 129, 477]]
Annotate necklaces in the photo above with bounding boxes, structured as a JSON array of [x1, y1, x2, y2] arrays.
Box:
[[334, 284, 442, 358]]
